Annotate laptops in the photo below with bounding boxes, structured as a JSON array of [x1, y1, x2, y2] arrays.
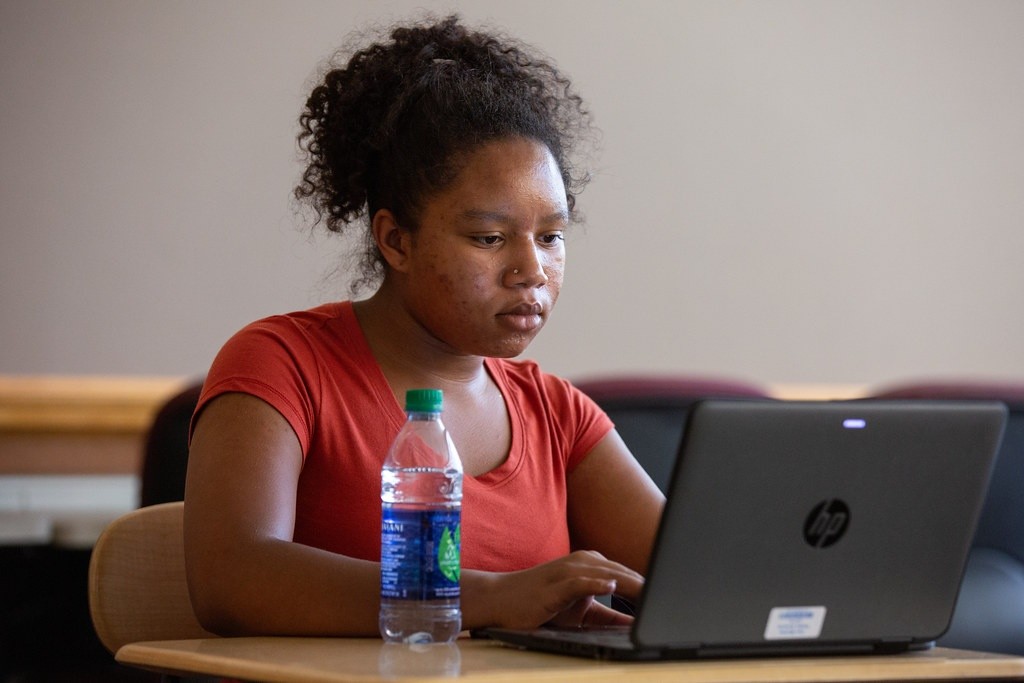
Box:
[[465, 400, 1004, 661]]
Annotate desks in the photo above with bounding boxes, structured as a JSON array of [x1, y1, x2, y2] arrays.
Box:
[[114, 632, 1024, 683]]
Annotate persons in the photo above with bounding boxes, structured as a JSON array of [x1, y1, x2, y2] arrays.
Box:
[[184, 16, 666, 640]]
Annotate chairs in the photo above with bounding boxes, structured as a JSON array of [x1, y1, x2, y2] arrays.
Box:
[[86, 502, 218, 656]]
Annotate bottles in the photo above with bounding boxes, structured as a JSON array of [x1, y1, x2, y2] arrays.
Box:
[[378, 389, 462, 646]]
